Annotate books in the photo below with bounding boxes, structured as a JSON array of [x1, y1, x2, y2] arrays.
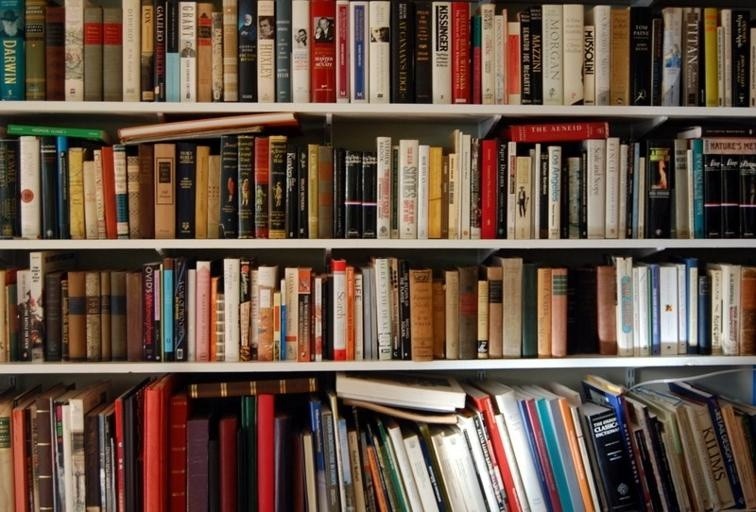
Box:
[[1, 249, 756, 363], [1, 375, 756, 511], [1, 114, 755, 239], [1, 0, 756, 106]]
